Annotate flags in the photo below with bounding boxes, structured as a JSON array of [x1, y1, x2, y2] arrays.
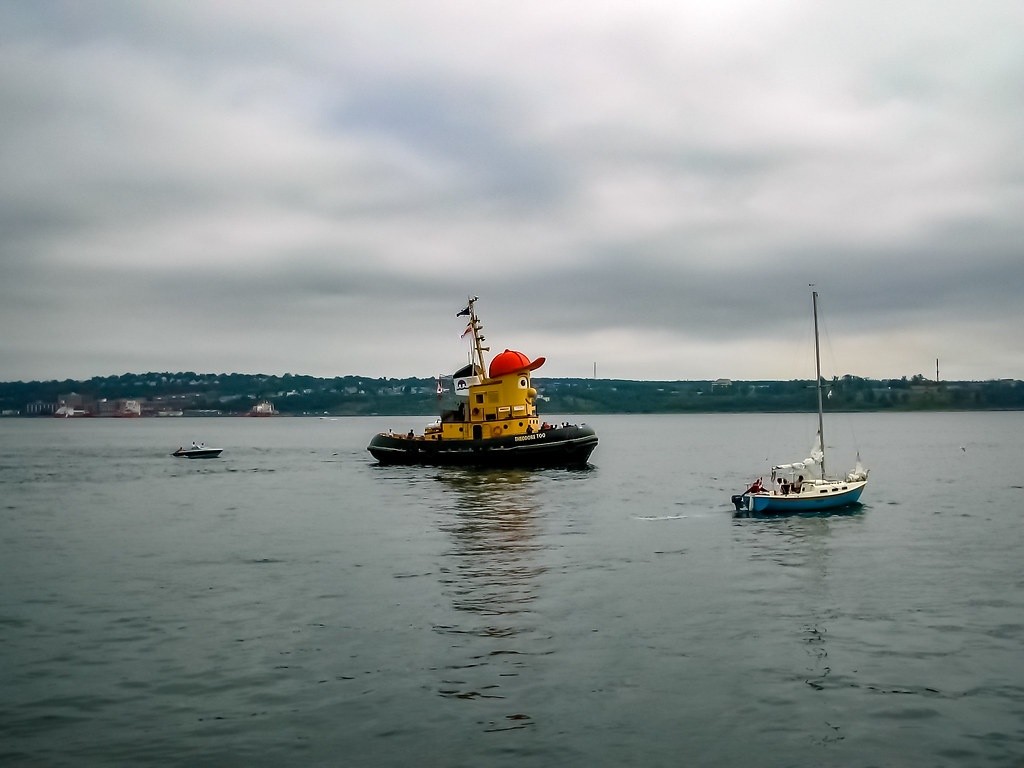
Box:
[[456, 306, 470, 317], [437, 379, 449, 394], [461, 323, 475, 342]]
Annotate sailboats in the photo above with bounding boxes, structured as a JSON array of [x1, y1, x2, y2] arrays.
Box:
[[729, 291, 870, 514]]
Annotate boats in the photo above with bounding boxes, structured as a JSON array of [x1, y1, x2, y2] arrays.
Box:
[[55, 407, 89, 417], [123, 400, 141, 417], [367, 295, 599, 471], [250, 405, 272, 416], [158, 411, 183, 418], [169, 441, 223, 459]]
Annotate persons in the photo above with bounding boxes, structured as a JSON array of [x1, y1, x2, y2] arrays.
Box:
[[191, 441, 205, 450], [526, 421, 566, 434], [408, 429, 414, 439], [388, 429, 395, 438], [776, 475, 804, 495]]
